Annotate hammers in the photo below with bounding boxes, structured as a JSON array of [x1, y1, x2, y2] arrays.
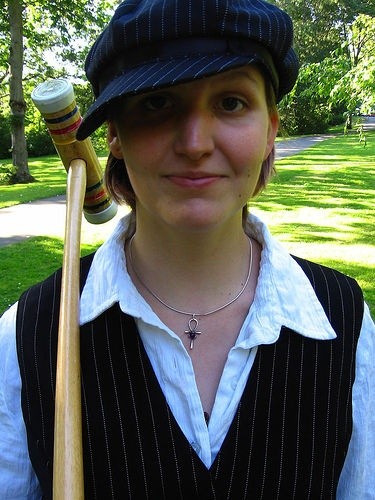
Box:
[[29, 78, 118, 500]]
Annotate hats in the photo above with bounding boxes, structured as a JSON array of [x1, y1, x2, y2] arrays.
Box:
[[75, 0, 299, 141]]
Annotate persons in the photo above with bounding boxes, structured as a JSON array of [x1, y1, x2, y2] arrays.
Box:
[[0, 0, 374, 500]]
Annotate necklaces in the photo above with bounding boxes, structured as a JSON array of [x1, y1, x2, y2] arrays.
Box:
[[128, 233, 252, 350]]
